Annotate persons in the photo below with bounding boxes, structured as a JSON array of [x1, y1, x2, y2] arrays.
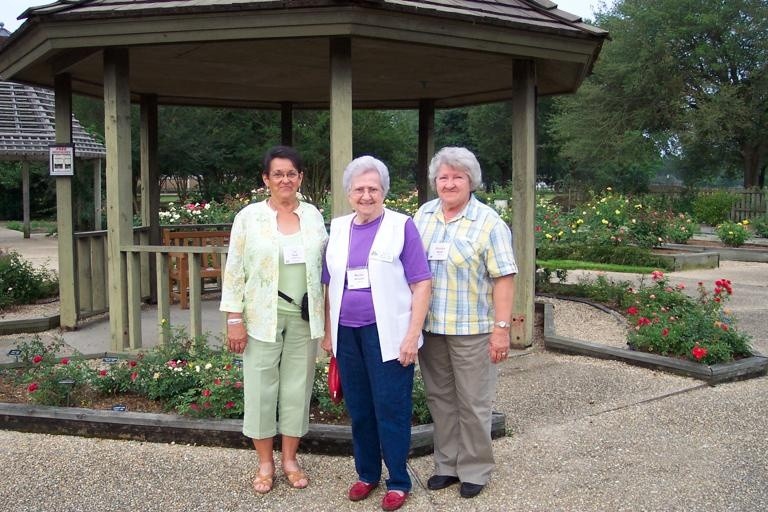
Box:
[[216, 144, 333, 494], [411, 146, 517, 500], [321, 155, 434, 511]]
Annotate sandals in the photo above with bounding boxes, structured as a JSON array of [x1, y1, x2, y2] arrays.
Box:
[[253, 458, 276, 494], [280, 459, 308, 489]]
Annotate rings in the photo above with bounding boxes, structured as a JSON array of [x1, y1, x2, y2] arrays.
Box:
[[501, 351, 506, 354], [409, 359, 414, 362]]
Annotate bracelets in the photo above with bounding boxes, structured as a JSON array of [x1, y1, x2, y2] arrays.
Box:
[[227, 318, 243, 325]]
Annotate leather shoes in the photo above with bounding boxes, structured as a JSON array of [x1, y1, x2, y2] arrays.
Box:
[[428, 476, 458, 489], [460, 482, 483, 498]]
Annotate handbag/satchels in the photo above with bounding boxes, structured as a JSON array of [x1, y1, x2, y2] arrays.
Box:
[[328, 357, 342, 403], [301, 293, 309, 321]]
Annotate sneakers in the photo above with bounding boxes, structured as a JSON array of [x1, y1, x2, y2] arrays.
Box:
[[350, 481, 378, 500], [382, 489, 407, 511]]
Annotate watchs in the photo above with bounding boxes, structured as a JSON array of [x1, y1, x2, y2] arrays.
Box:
[[495, 320, 511, 328]]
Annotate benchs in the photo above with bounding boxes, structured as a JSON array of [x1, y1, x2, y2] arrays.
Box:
[[161, 228, 234, 309]]
